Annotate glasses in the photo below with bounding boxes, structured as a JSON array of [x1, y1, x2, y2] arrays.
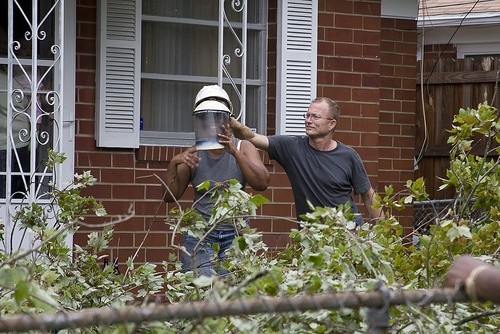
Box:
[[303, 113, 333, 120]]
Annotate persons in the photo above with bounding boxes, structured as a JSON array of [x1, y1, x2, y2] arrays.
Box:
[[0, 26, 44, 199], [161, 85, 270, 277], [230, 96, 386, 231]]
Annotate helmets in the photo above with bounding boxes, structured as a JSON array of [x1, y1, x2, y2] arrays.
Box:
[[191, 85, 234, 115]]
